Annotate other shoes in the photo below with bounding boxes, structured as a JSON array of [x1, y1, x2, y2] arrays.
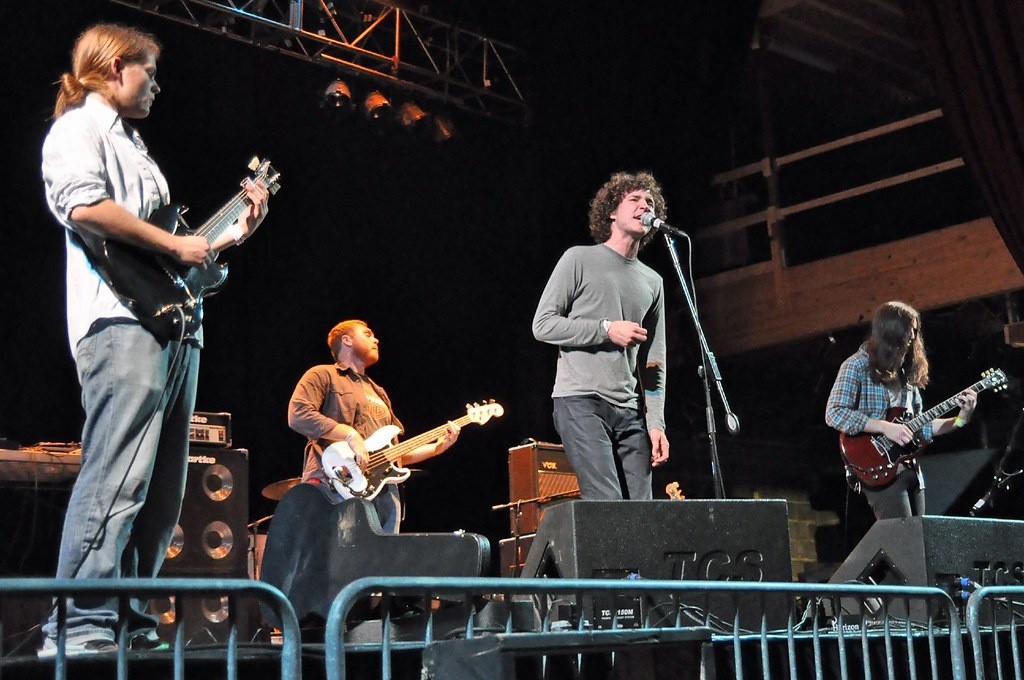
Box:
[[127, 628, 170, 652], [35, 639, 118, 657]]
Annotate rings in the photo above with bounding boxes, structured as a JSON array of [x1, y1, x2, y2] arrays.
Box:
[[449, 438, 454, 441]]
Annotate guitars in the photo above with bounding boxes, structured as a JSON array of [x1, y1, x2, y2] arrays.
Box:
[[664, 478, 687, 502], [838, 367, 1010, 488], [316, 397, 506, 503], [85, 155, 281, 340]]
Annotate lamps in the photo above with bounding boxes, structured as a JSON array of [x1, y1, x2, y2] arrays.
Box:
[[325, 79, 457, 147]]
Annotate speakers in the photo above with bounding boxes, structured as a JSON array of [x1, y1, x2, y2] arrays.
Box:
[[137, 447, 250, 648], [817, 514, 1024, 626], [0, 458, 88, 652], [497, 442, 798, 635]]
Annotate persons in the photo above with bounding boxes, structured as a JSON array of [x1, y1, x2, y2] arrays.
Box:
[[289, 320, 461, 533], [826, 301, 978, 520], [41, 24, 268, 654], [532, 171, 670, 499]]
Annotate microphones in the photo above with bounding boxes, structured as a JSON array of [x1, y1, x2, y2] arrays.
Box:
[[640, 211, 688, 238], [970, 471, 1021, 517]]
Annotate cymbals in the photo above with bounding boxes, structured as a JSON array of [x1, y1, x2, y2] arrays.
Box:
[[262, 477, 321, 500]]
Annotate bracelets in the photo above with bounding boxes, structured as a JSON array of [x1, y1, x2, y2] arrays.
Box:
[[231, 225, 246, 246], [955, 416, 963, 427], [604, 321, 611, 332]]
